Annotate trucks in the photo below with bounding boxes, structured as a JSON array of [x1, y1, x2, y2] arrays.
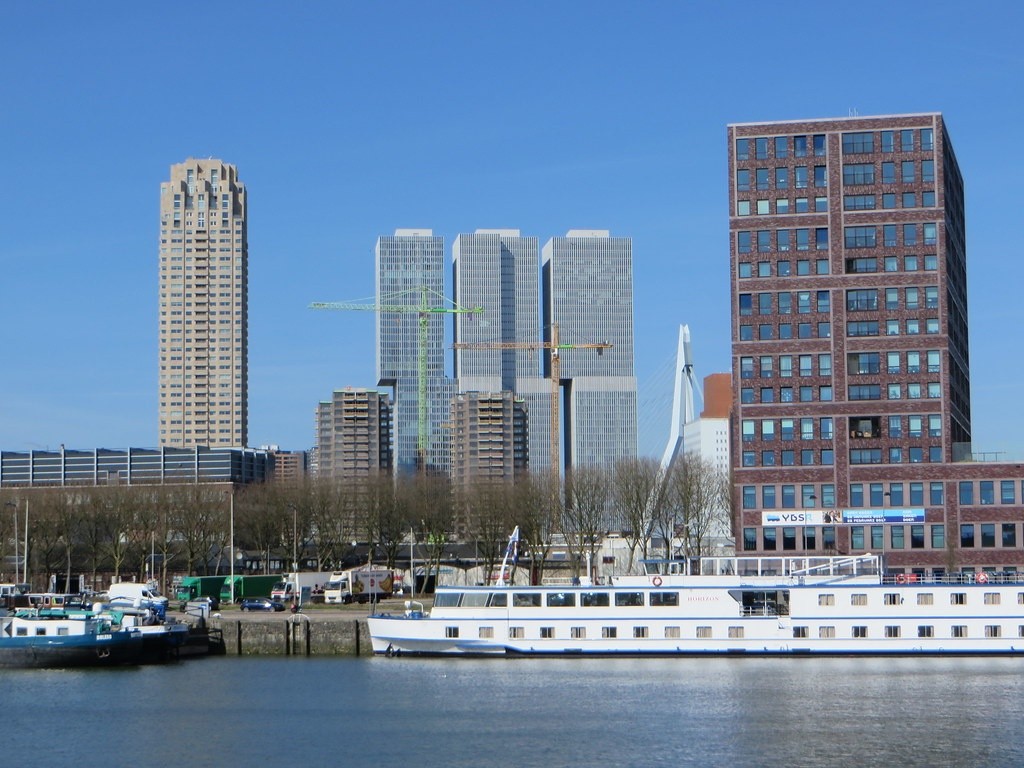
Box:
[[220, 575, 283, 606], [323, 569, 395, 604], [176, 575, 227, 604], [270, 570, 333, 605]]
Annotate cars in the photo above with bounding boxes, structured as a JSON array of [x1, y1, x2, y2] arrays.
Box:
[[192, 595, 219, 612], [240, 597, 285, 612], [64, 580, 170, 618]]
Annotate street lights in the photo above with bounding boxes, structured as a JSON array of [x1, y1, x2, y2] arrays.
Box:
[[286, 502, 299, 610], [6, 501, 19, 584], [20, 497, 29, 584], [882, 492, 893, 556], [804, 495, 818, 575], [223, 490, 234, 605]]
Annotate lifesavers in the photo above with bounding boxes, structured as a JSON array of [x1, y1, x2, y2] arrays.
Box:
[[976, 572, 988, 584], [896, 573, 908, 584], [653, 576, 663, 587]]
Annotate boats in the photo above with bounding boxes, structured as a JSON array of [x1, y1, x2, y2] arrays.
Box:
[[0, 592, 210, 666], [366, 522, 1024, 658]]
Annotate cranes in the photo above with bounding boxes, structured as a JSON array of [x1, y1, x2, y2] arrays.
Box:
[[308, 276, 483, 546], [448, 321, 614, 533]]
